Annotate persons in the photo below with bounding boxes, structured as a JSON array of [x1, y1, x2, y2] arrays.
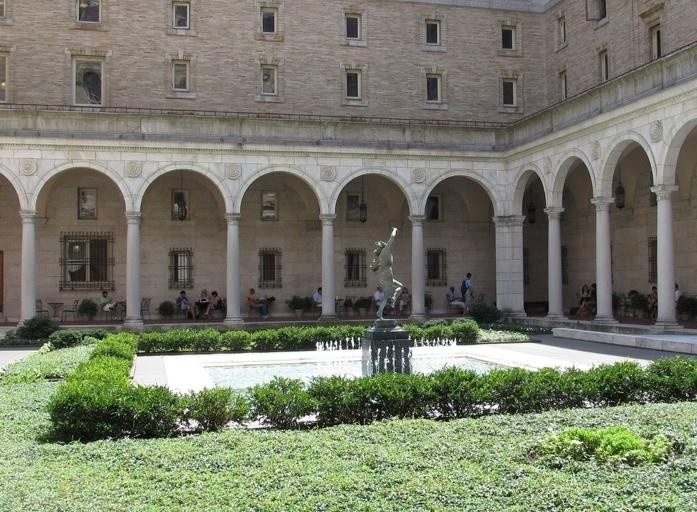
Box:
[[647, 286, 657, 320], [100, 291, 118, 322], [462, 273, 478, 317], [203, 291, 222, 317], [247, 288, 269, 319], [374, 285, 388, 319], [398, 287, 412, 318], [577, 284, 590, 316], [674, 283, 683, 310], [176, 291, 198, 322], [369, 224, 405, 322], [313, 287, 323, 309], [447, 285, 468, 317], [580, 283, 596, 317]]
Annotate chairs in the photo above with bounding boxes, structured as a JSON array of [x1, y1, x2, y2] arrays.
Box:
[[309, 291, 487, 318], [575, 290, 684, 323], [35, 298, 153, 322]]
